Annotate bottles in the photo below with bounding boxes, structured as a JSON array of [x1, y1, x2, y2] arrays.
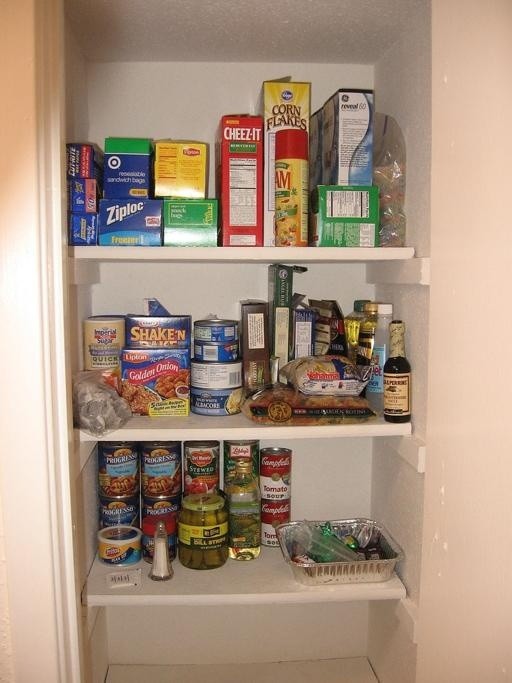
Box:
[[226, 457, 261, 563], [273, 128, 312, 246], [343, 299, 411, 423], [148, 520, 176, 583]]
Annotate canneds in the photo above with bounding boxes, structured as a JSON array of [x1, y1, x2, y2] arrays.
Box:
[[190, 318, 244, 416], [97, 440, 292, 568]]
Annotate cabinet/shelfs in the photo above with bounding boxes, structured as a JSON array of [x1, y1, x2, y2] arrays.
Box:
[[45, 0, 430, 683]]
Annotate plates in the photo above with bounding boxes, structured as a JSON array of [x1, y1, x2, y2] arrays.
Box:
[[121, 378, 162, 403]]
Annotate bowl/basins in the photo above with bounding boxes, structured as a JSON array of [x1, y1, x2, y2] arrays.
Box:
[[174, 385, 189, 399]]
